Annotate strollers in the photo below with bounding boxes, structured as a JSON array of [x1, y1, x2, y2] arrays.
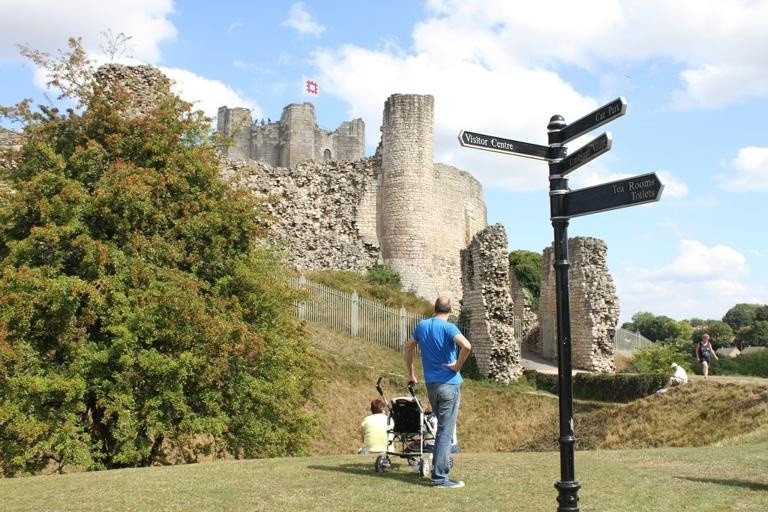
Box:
[[372, 376, 460, 480]]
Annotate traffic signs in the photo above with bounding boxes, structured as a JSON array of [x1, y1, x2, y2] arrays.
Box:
[[562, 172, 664, 220], [558, 95, 628, 149], [457, 129, 552, 163], [560, 131, 612, 179]]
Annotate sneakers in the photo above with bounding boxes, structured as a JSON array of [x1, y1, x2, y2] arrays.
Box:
[[433, 479, 465, 487]]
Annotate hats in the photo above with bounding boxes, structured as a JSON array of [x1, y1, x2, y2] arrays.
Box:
[[671, 363, 677, 368]]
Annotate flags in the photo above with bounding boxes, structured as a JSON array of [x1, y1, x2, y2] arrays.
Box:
[[307, 80, 318, 95]]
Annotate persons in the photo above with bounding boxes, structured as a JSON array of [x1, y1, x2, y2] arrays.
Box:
[[424, 410, 460, 454], [404, 297, 472, 489], [657, 363, 688, 393], [255, 119, 258, 124], [696, 334, 718, 378], [261, 119, 264, 125], [268, 118, 271, 123], [357, 399, 394, 455]]
